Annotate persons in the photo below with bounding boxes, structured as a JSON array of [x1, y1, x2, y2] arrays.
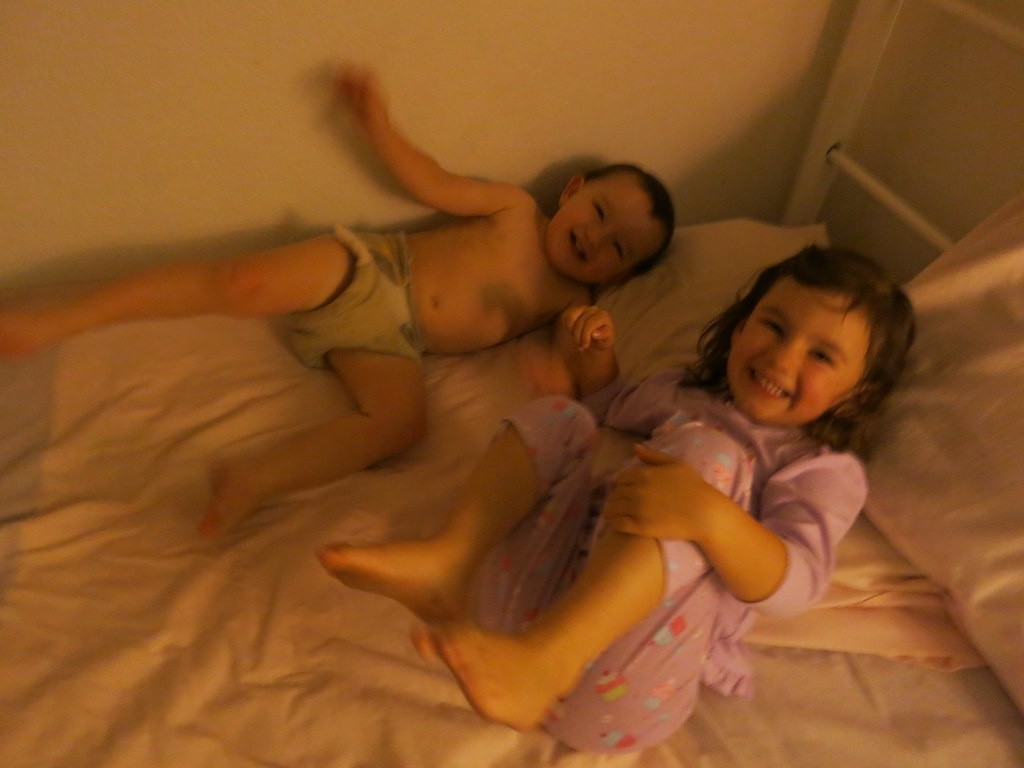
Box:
[[0, 60, 676, 546], [314, 244, 920, 756]]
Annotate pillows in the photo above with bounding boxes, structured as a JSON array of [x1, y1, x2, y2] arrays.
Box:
[[853, 190, 1024, 721], [590, 216, 830, 388]]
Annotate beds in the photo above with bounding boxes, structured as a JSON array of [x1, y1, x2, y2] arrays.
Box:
[[0, 1, 1024, 765]]
